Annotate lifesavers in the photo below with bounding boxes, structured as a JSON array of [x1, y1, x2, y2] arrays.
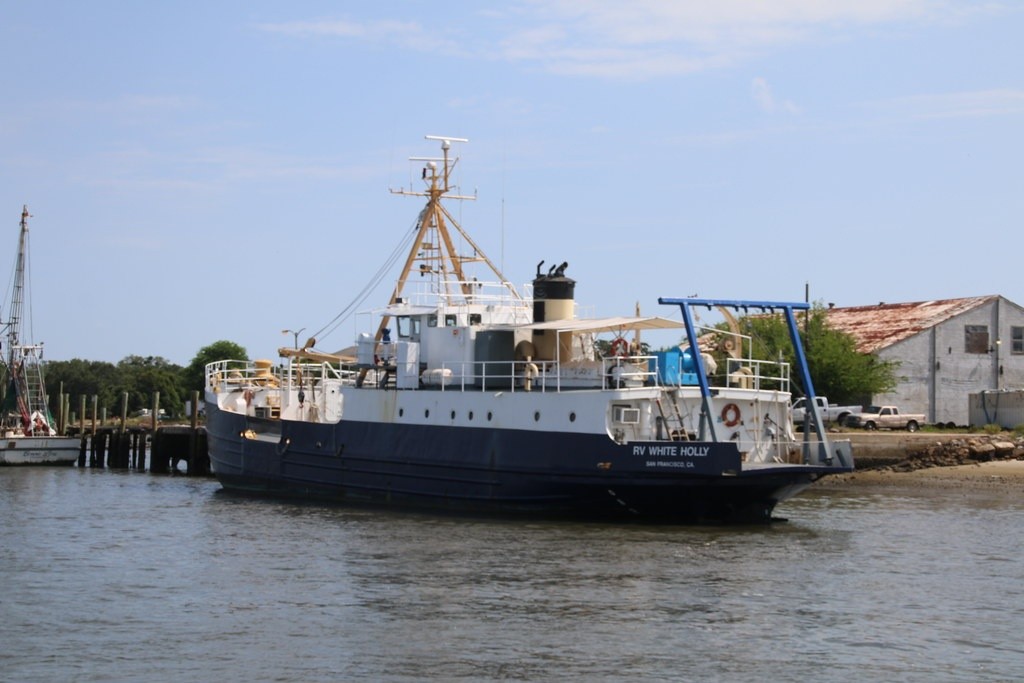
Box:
[[610, 337, 629, 360], [722, 403, 742, 427]]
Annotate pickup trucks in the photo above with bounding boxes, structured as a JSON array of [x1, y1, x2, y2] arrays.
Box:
[[793, 396, 863, 428], [847, 407, 927, 432]]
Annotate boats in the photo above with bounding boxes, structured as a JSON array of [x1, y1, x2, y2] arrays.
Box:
[[203, 136, 846, 526]]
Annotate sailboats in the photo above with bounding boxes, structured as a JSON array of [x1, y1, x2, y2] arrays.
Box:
[[0, 205, 84, 468]]
